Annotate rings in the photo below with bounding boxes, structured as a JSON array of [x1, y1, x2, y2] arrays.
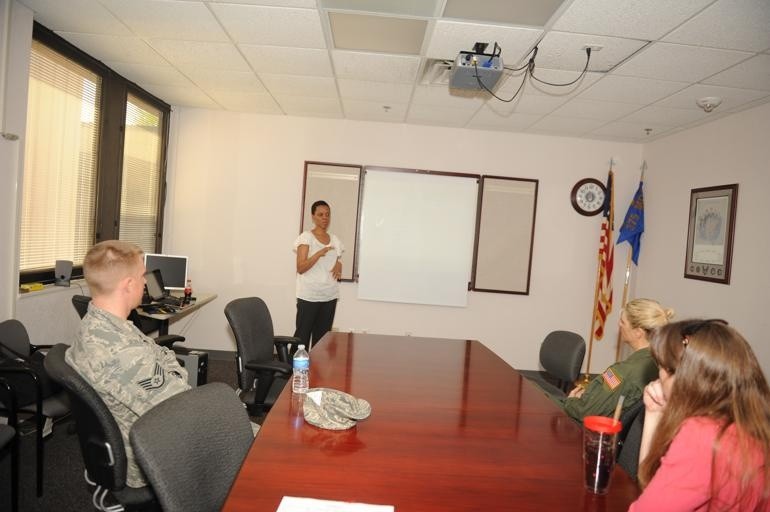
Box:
[[337, 272, 341, 274]]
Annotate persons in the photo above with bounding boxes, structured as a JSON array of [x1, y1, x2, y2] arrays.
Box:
[[628, 318, 770, 512], [565, 297, 675, 422], [65, 240, 261, 489], [289, 200, 345, 367]]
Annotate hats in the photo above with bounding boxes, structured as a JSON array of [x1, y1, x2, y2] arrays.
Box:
[[298, 384, 374, 433]]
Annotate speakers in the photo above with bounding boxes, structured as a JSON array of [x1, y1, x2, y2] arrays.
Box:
[[55, 260, 73, 287]]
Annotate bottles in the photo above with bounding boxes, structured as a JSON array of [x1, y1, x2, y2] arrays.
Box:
[[291, 344, 311, 395], [184, 280, 193, 305]]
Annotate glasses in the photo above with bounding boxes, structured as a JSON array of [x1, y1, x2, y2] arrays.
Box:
[[678, 317, 731, 350]]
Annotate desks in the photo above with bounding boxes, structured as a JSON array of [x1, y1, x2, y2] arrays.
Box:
[[220, 330, 643, 512], [135, 293, 217, 337]]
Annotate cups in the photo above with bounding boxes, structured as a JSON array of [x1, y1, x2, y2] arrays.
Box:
[[583, 415, 624, 496]]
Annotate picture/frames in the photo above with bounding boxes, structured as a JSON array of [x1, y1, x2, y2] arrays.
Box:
[[684, 183, 739, 285]]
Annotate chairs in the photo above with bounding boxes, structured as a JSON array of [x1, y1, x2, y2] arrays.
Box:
[[0, 319, 74, 498], [44, 344, 159, 512], [71, 295, 185, 353], [617, 406, 644, 483], [128, 381, 255, 512], [520, 331, 586, 401], [0, 376, 18, 512], [616, 399, 644, 430], [224, 298, 302, 416]]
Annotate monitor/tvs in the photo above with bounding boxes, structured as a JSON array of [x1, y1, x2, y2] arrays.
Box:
[[144, 253, 188, 305]]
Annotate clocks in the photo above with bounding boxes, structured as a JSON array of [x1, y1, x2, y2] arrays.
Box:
[[571, 178, 607, 216]]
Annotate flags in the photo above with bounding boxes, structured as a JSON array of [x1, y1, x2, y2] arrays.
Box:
[[594, 170, 614, 340], [617, 181, 644, 265]]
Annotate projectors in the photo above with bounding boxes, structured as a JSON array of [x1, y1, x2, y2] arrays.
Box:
[[449, 52, 504, 90]]
